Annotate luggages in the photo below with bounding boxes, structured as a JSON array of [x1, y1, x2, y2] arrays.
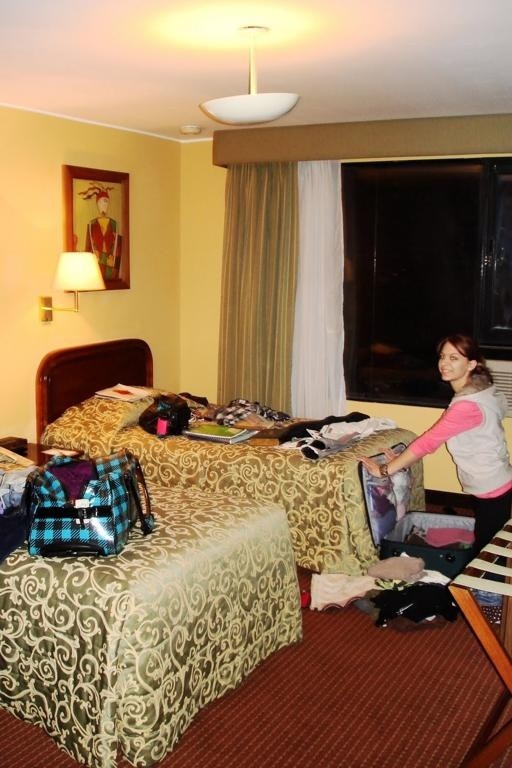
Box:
[[358, 442, 479, 580]]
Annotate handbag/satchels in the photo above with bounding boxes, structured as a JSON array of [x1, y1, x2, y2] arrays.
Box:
[[139, 393, 190, 437], [22, 447, 155, 558]]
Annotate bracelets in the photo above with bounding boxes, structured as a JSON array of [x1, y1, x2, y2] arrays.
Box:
[[379, 464, 388, 477]]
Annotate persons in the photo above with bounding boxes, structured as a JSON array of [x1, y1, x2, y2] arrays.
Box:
[[355, 334, 512, 583]]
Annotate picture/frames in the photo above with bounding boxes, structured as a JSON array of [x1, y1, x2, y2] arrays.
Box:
[[62, 164, 130, 292]]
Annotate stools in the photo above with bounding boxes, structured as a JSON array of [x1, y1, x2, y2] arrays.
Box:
[[446, 517, 512, 768]]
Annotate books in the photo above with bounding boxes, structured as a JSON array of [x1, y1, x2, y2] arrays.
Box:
[[182, 424, 260, 444]]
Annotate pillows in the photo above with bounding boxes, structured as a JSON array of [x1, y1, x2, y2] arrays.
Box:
[[40, 386, 204, 458]]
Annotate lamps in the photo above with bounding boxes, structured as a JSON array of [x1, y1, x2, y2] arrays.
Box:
[[199, 27, 300, 125], [39, 252, 107, 321]]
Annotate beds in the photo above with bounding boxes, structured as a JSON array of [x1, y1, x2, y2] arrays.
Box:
[[34, 339, 426, 572], [0, 478, 303, 768]]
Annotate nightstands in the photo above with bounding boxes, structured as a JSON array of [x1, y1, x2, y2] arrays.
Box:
[[0, 443, 84, 467]]
